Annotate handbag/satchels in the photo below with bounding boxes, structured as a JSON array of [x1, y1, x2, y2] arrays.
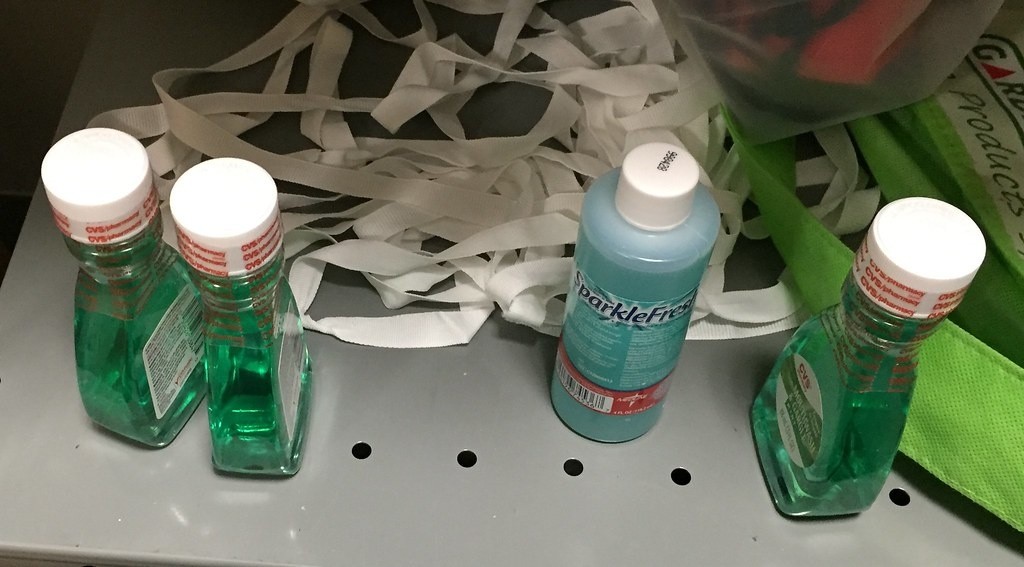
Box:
[[718, 0, 1024, 533]]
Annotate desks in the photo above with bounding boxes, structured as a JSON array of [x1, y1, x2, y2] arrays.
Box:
[[0, 0, 1024, 567]]
